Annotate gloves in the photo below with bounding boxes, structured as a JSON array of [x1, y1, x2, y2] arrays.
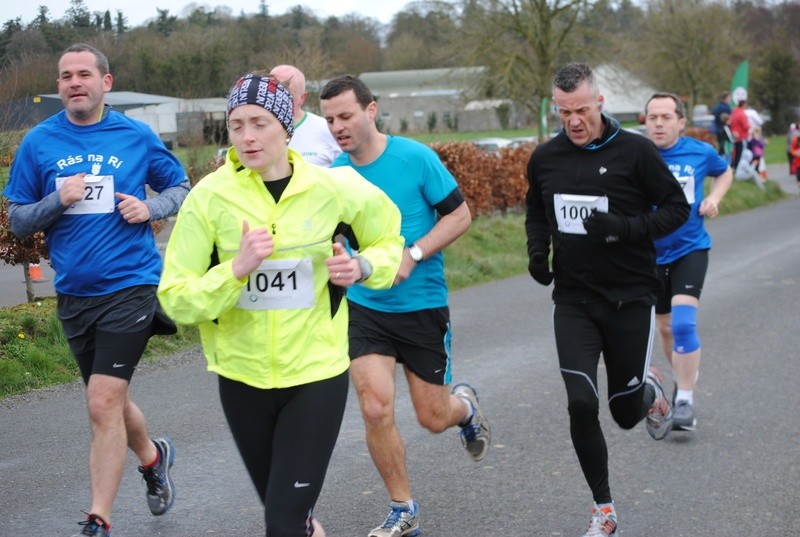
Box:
[[582, 209, 632, 245], [528, 255, 554, 287]]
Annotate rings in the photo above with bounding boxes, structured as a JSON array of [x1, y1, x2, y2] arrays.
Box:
[[126, 214, 130, 219], [336, 272, 340, 279]]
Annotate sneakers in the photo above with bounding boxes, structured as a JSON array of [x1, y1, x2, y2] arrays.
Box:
[[137, 437, 175, 516], [368, 498, 419, 537], [583, 500, 619, 537], [451, 383, 491, 461], [671, 381, 678, 408], [643, 366, 673, 440], [670, 399, 697, 431], [70, 509, 111, 537]]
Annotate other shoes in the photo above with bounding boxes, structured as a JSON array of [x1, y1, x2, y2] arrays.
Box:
[[311, 519, 326, 537]]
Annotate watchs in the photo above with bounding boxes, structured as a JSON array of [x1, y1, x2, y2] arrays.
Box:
[[407, 242, 424, 265]]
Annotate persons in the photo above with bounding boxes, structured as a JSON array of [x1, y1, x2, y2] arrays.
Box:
[[523, 63, 691, 537], [709, 91, 800, 191], [320, 75, 492, 537], [269, 65, 343, 168], [1, 42, 192, 537], [645, 92, 734, 431], [156, 68, 406, 537]]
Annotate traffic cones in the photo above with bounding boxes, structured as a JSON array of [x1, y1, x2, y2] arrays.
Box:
[[22, 261, 53, 283]]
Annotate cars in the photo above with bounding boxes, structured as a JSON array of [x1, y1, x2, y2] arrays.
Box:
[[473, 134, 536, 155], [689, 113, 718, 132]]
